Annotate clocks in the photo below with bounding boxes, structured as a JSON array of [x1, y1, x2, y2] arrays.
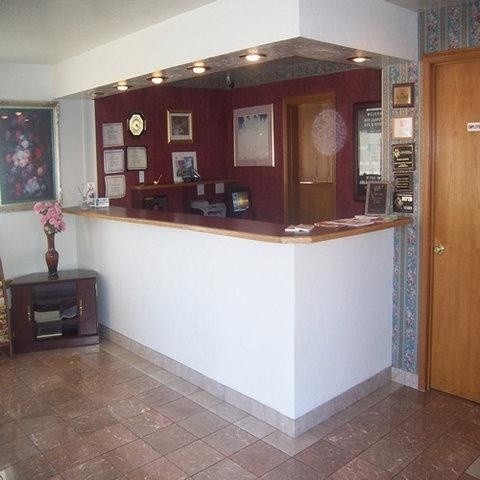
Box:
[[126, 110, 147, 139]]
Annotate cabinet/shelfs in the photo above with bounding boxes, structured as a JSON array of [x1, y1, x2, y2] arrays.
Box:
[[7, 268, 102, 356]]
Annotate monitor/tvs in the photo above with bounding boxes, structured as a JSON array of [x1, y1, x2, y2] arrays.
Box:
[[143, 195, 170, 212], [228, 185, 252, 216]]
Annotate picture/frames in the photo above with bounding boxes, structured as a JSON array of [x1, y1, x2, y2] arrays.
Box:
[[99, 118, 149, 201], [352, 100, 383, 202], [232, 103, 277, 169], [164, 107, 194, 146], [170, 151, 198, 183], [0, 97, 62, 216], [389, 80, 417, 193]]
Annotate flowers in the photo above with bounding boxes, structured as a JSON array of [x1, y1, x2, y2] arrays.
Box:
[[33, 198, 64, 235]]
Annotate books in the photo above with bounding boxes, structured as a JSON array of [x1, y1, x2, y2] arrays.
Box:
[[284, 214, 379, 234], [33, 303, 79, 340]]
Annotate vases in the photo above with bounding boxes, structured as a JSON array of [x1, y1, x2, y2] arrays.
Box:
[[45, 230, 59, 276]]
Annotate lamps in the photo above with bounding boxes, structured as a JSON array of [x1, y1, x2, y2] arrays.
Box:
[[145, 75, 169, 85], [238, 53, 267, 62], [186, 65, 210, 75], [112, 83, 133, 91], [345, 54, 372, 64]]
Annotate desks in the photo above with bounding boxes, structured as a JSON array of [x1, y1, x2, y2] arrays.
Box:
[[131, 178, 237, 216]]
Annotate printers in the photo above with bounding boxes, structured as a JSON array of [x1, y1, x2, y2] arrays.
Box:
[[191, 200, 227, 218]]
